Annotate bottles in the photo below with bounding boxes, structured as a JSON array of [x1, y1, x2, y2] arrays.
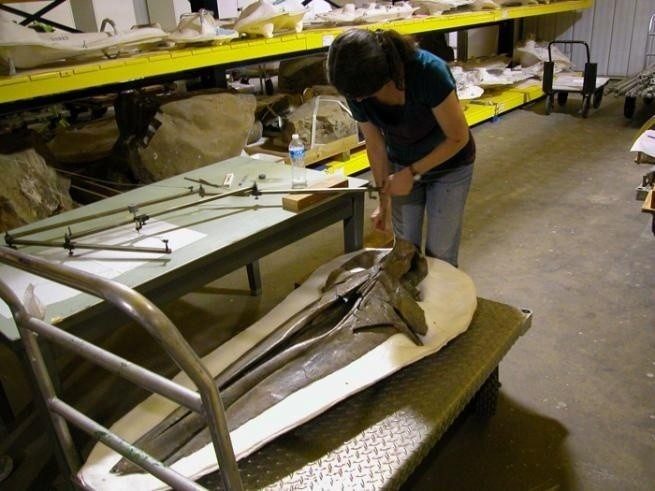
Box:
[[288, 134, 307, 185]]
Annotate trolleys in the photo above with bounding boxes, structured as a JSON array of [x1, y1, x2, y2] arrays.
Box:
[[543, 40, 609, 117], [0, 246, 533, 490], [624, 14, 655, 118]]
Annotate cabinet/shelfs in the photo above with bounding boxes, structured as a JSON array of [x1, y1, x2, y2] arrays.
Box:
[[0, 0, 596, 212]]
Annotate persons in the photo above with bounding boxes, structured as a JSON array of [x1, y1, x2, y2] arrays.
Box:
[[326, 26, 476, 268]]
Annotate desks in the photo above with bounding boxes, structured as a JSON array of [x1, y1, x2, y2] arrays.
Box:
[[0, 157, 368, 491]]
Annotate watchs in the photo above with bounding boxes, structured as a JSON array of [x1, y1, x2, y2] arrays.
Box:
[[407, 164, 422, 183]]
